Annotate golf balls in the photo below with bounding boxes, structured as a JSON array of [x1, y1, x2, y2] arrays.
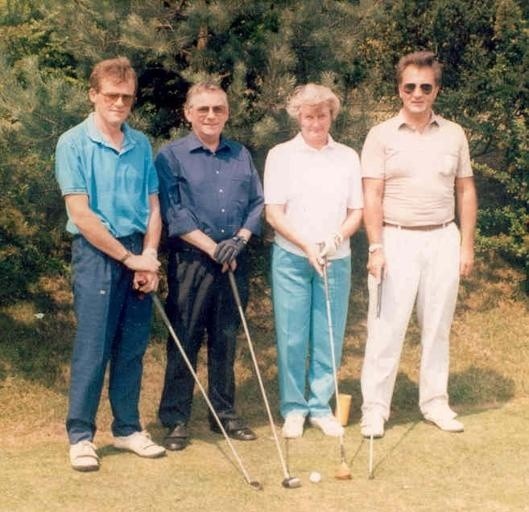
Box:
[[310, 472, 320, 482]]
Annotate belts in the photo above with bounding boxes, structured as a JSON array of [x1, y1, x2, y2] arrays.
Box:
[[383, 217, 456, 233]]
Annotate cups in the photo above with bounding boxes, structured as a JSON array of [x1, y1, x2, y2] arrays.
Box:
[[334, 391, 353, 428]]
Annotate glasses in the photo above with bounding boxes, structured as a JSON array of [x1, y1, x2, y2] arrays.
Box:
[[403, 83, 436, 94], [99, 90, 136, 107]]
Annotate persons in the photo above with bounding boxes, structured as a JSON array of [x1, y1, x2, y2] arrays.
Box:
[[52, 53, 168, 472], [357, 49, 478, 439], [155, 78, 265, 451], [262, 79, 364, 441]]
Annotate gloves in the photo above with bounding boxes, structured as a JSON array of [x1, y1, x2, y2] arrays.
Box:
[[213, 235, 249, 266], [315, 230, 343, 260]]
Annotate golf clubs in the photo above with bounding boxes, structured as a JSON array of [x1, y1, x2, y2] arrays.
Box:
[[139, 279, 263, 492], [368, 268, 385, 480], [228, 267, 301, 489], [322, 258, 352, 478]]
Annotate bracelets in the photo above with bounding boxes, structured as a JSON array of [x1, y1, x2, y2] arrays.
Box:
[[367, 243, 383, 253], [120, 248, 133, 264]]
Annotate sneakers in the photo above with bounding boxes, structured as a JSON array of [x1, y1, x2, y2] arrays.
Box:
[[69, 439, 100, 472], [307, 409, 345, 438], [112, 429, 167, 458], [163, 423, 189, 452], [423, 409, 465, 433], [359, 412, 385, 438], [280, 412, 305, 439], [209, 409, 257, 440]]
